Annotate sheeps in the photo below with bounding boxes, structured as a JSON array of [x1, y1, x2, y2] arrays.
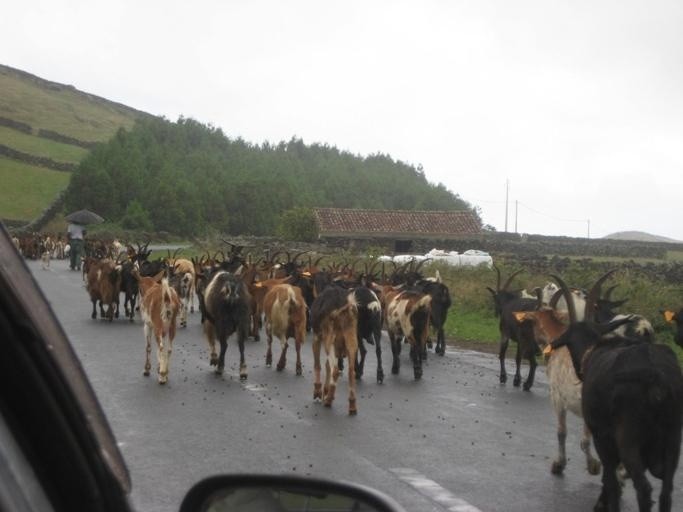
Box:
[[485, 261, 549, 391], [347, 286, 386, 383], [308, 286, 359, 416], [12, 233, 71, 260], [190, 254, 251, 382], [514, 287, 653, 478], [133, 264, 184, 384], [404, 257, 452, 358], [252, 270, 308, 376], [521, 281, 656, 340], [83, 232, 451, 355], [379, 264, 430, 381], [550, 267, 683, 512]]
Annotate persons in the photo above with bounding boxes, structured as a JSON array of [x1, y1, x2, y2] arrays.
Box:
[[67, 220, 86, 270]]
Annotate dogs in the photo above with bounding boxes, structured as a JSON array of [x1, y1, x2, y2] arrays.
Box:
[[40, 250, 51, 270]]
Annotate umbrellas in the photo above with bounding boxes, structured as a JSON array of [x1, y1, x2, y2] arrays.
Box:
[[64, 209, 104, 226]]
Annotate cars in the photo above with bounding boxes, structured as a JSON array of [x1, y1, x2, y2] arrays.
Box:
[[0, 220, 402, 512]]
